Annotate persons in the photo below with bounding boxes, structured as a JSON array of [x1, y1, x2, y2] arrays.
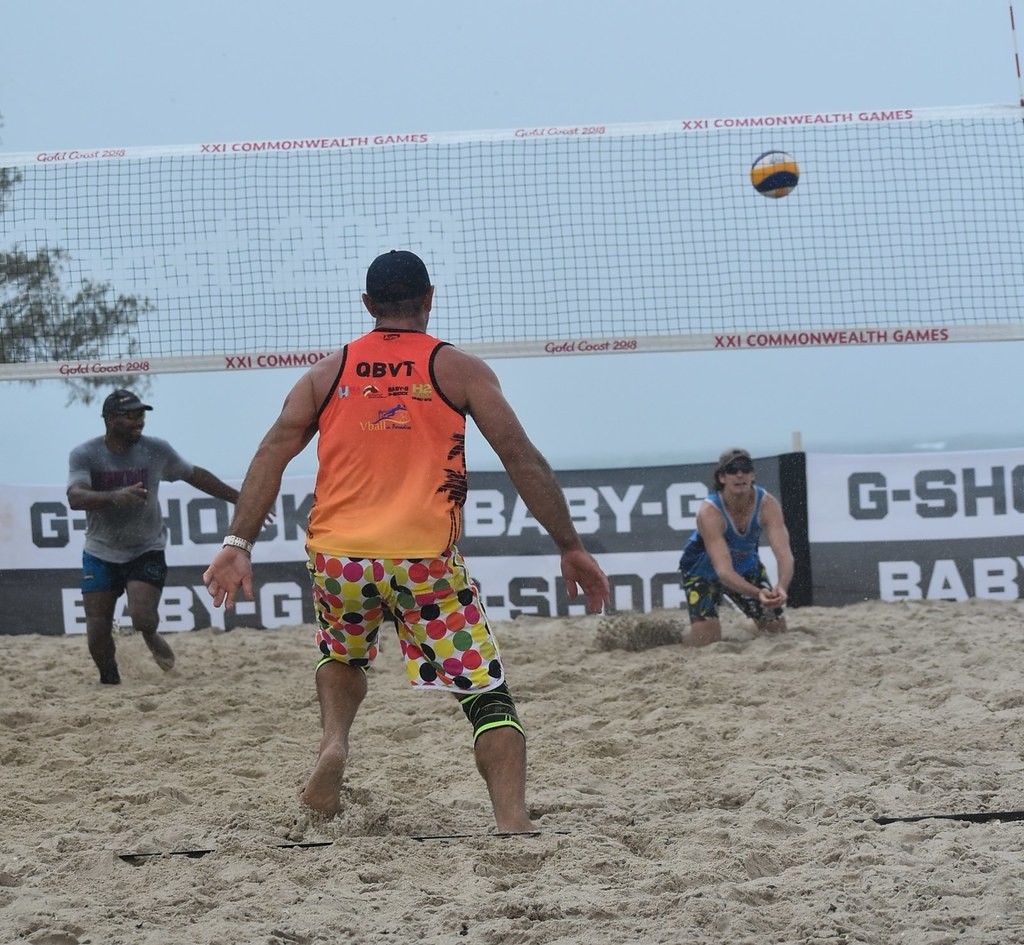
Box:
[[679, 449, 793, 647], [67, 391, 276, 686], [203, 249, 611, 836]]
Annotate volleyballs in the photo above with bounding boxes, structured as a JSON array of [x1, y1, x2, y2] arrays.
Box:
[[751, 148, 801, 199]]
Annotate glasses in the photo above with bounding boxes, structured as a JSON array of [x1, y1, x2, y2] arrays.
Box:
[[726, 464, 754, 474], [121, 410, 146, 420]]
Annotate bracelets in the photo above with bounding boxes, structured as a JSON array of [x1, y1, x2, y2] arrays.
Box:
[[223, 535, 253, 554]]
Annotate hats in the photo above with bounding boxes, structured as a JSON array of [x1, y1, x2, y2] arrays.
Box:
[[719, 447, 752, 469], [366, 248, 431, 303], [102, 388, 154, 414]]
